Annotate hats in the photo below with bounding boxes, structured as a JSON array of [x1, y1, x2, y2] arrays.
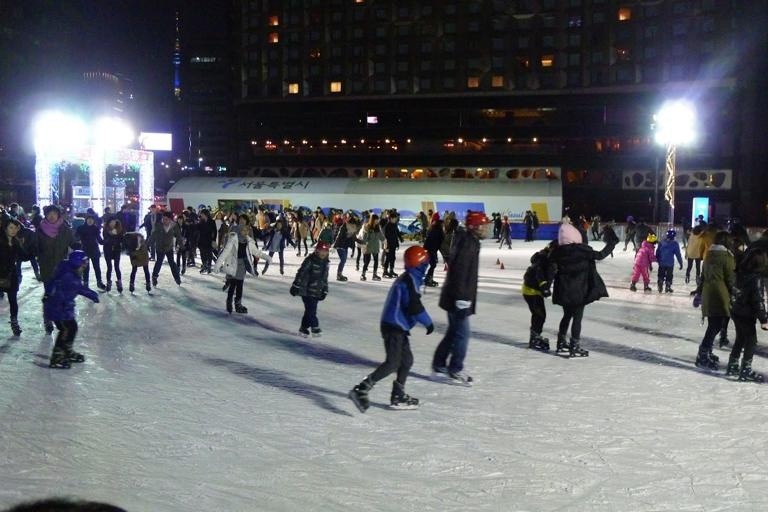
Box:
[[431, 212, 439, 223], [317, 241, 329, 251]]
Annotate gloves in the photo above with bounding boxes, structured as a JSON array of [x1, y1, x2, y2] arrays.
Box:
[[427, 323, 433, 334], [541, 279, 552, 297], [319, 293, 325, 300], [679, 264, 682, 269], [290, 286, 299, 296], [693, 295, 701, 307]]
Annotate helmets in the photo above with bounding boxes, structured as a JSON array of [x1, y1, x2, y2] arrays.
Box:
[[647, 234, 657, 242], [666, 229, 675, 237], [465, 212, 491, 230], [405, 246, 429, 268]]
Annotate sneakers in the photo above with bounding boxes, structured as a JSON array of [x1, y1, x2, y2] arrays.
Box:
[[433, 366, 471, 382], [686, 276, 700, 284], [299, 327, 321, 334], [262, 269, 284, 274], [296, 251, 308, 256]]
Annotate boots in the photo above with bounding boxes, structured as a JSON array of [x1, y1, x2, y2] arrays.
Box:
[[43, 314, 54, 335], [391, 381, 418, 405], [51, 343, 83, 365], [175, 259, 211, 284], [424, 275, 438, 286], [353, 376, 376, 409], [227, 296, 246, 312], [9, 315, 21, 337], [630, 281, 673, 292], [529, 329, 588, 355], [362, 271, 397, 279], [338, 271, 347, 279], [729, 356, 762, 379], [97, 272, 158, 292], [696, 346, 719, 369]]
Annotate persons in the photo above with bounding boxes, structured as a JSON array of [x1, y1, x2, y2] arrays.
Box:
[[346, 247, 433, 415], [521, 238, 560, 351], [42, 247, 99, 365], [553, 223, 615, 356], [431, 211, 496, 384], [564, 206, 767, 382], [1, 202, 539, 338]]
